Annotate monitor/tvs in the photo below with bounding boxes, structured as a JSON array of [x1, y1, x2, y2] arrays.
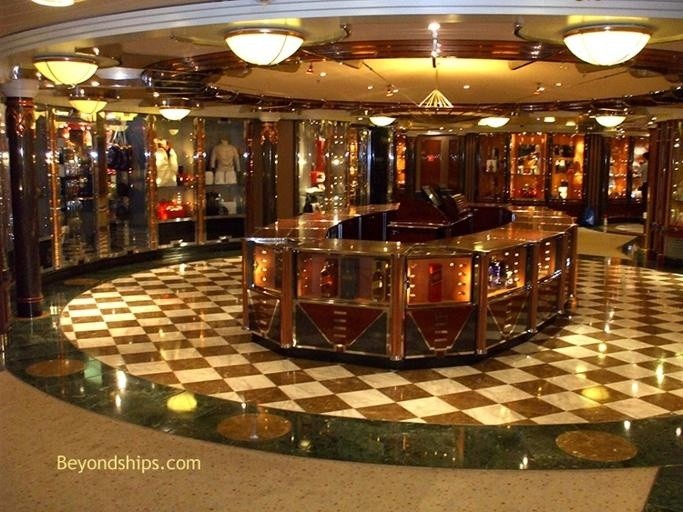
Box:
[[421, 184, 442, 206]]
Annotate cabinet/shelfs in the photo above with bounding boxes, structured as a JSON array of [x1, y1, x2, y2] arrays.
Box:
[[242, 202, 580, 370]]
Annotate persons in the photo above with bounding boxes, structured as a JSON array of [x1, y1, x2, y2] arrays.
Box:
[[152, 140, 179, 188], [631, 151, 649, 212], [210, 138, 241, 184]]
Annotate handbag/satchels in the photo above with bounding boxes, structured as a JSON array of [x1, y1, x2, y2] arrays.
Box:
[[206, 192, 237, 215], [155, 198, 194, 220], [60, 174, 93, 197], [107, 129, 136, 172]]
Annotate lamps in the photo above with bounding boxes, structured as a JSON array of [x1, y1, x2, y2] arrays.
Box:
[[31, 22, 654, 128]]
